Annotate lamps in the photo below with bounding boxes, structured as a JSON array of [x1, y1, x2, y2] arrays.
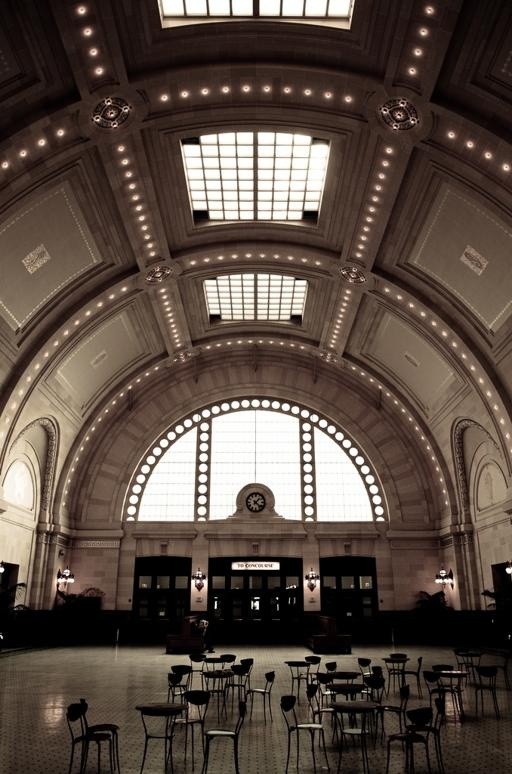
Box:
[[63, 571, 75, 586], [56, 566, 65, 586], [436, 572, 446, 584], [193, 568, 207, 592], [443, 570, 455, 586], [307, 567, 321, 593]]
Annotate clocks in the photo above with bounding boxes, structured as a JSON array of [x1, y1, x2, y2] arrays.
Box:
[[246, 492, 266, 513]]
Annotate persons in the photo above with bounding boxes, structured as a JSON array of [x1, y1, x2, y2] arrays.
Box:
[[191, 621, 214, 653]]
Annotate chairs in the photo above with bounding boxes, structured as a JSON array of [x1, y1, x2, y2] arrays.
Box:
[[66, 699, 121, 773], [136, 655, 253, 773], [244, 671, 275, 726], [422, 648, 512, 723], [280, 653, 444, 773]]
